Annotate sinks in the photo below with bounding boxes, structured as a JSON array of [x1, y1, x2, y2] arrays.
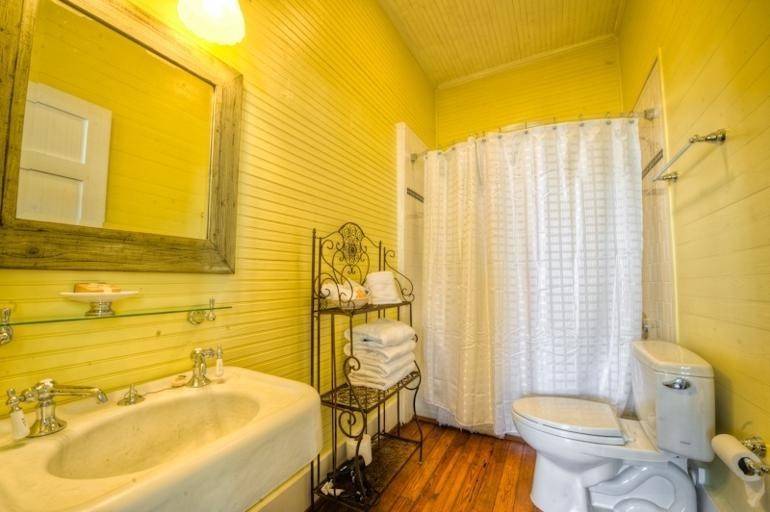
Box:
[[40, 385, 266, 486]]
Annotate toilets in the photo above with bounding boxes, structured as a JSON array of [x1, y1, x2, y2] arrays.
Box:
[[512, 343, 718, 512]]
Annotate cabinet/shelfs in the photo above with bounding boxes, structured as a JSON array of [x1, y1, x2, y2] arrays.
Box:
[[310, 220, 424, 512]]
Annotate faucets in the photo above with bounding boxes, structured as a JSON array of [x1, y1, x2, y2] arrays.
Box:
[[3, 379, 107, 438], [191, 345, 223, 387]]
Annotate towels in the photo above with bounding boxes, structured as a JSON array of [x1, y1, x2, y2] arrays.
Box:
[[321, 266, 416, 390]]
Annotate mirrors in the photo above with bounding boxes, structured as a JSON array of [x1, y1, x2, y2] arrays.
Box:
[[0, 0, 243, 274]]
